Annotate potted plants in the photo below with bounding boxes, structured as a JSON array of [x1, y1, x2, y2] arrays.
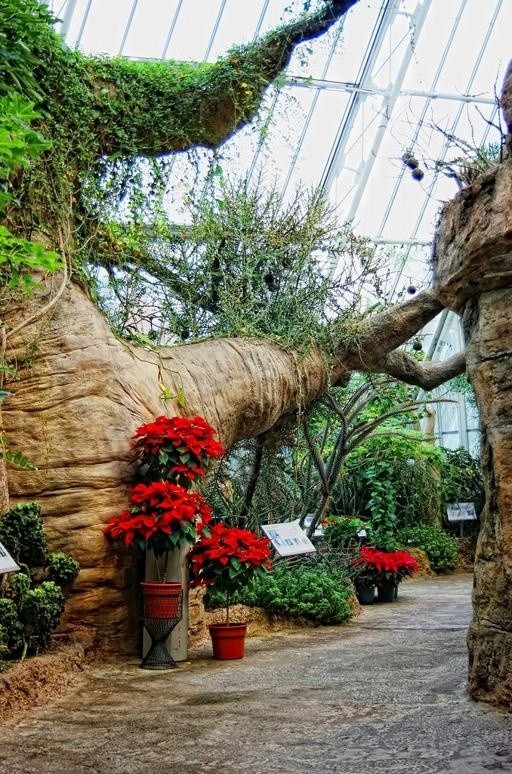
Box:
[[352, 547, 418, 604], [103, 480, 212, 617], [120, 416, 225, 662], [187, 523, 274, 661]]
